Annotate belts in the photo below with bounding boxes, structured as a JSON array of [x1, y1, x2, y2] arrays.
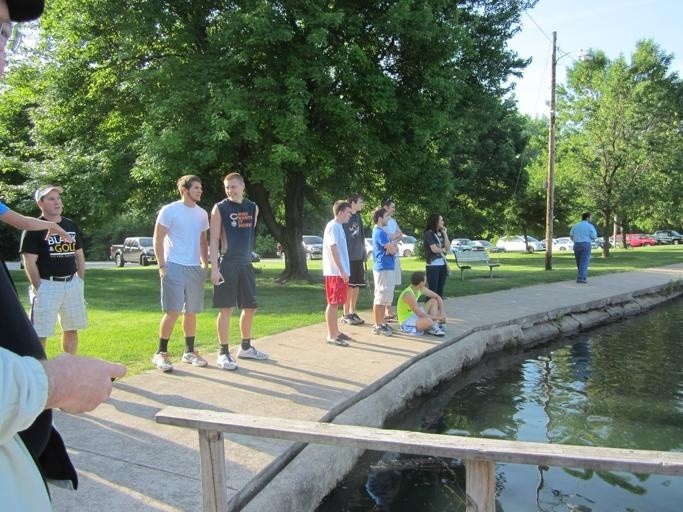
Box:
[[35, 276, 77, 283]]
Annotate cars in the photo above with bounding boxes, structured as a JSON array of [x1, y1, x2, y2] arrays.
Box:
[[616, 233, 656, 247], [596, 235, 615, 249], [452, 239, 482, 254], [550, 235, 577, 252], [474, 239, 503, 253]]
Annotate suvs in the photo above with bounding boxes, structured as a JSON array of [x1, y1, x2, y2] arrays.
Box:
[[497, 234, 543, 253], [397, 236, 421, 257], [359, 236, 377, 262]]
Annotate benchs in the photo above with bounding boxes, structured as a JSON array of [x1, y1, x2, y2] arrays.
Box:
[[452, 250, 500, 281]]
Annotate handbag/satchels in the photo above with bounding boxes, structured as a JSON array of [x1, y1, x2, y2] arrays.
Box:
[[430, 231, 450, 276]]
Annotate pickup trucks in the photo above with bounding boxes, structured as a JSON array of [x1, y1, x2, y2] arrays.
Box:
[[276, 236, 323, 259], [655, 229, 683, 244], [110, 236, 157, 267]]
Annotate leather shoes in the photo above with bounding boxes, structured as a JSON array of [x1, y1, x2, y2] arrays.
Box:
[[577, 279, 587, 283]]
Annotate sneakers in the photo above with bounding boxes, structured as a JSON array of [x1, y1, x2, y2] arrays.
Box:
[[384, 322, 395, 333], [340, 314, 359, 325], [151, 351, 173, 372], [236, 346, 269, 360], [182, 349, 208, 367], [371, 323, 392, 337], [428, 323, 445, 337], [350, 313, 364, 324], [217, 352, 238, 370]]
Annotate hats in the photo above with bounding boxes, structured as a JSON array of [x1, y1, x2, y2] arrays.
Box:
[[34, 184, 64, 202]]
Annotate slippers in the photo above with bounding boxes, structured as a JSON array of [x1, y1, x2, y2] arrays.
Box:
[[337, 333, 352, 341], [326, 337, 349, 347]]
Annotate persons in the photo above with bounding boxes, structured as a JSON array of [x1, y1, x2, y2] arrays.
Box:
[[19, 184, 89, 355], [210, 173, 270, 370], [153, 175, 207, 373], [569, 334, 593, 384], [322, 200, 352, 347], [424, 213, 450, 302], [0, 203, 75, 245], [372, 208, 399, 336], [0, 344, 127, 511], [339, 194, 366, 325], [569, 212, 598, 283], [397, 272, 448, 337], [382, 198, 403, 319]]
